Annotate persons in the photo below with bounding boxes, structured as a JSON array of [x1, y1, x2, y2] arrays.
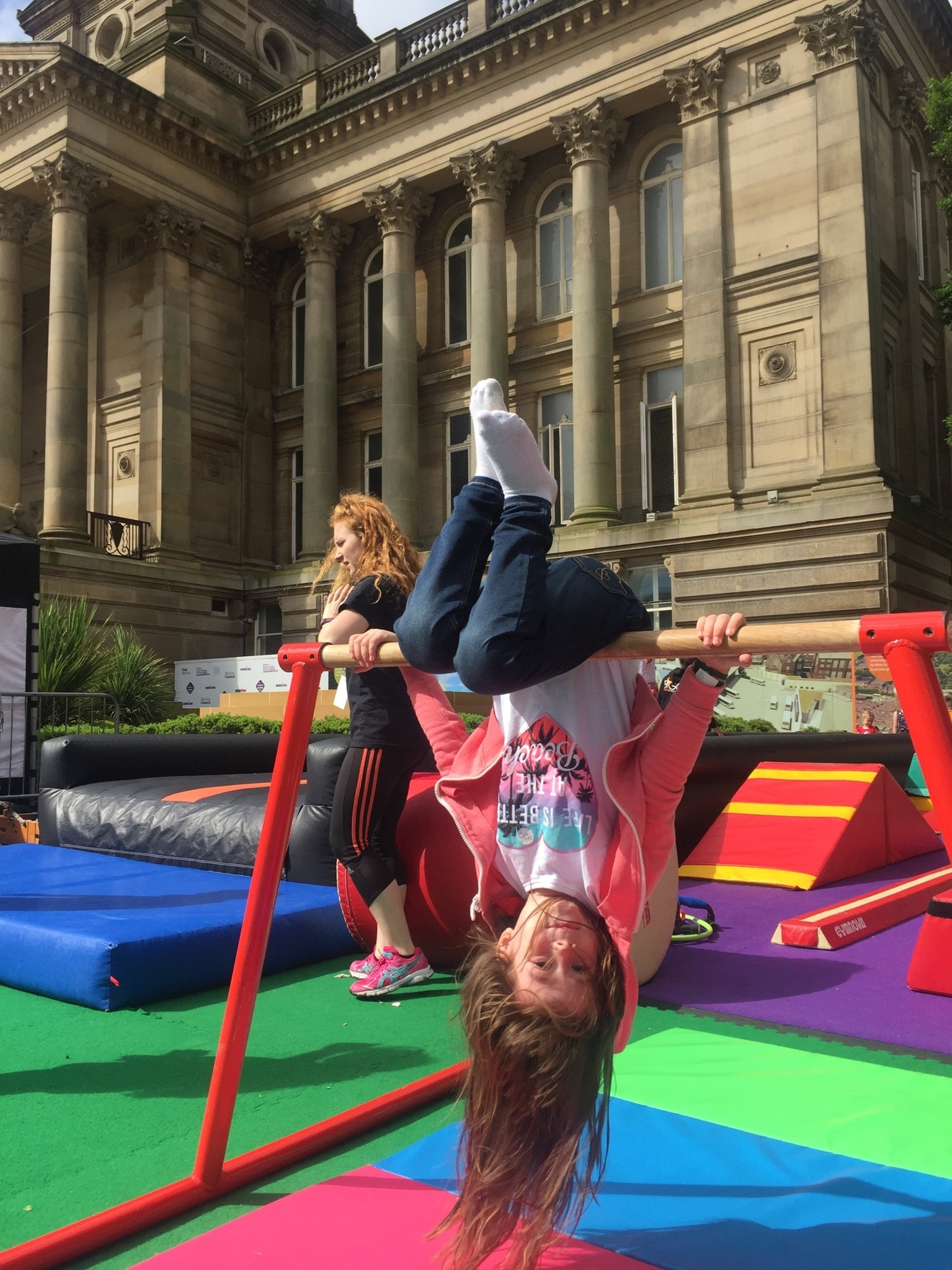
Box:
[[349, 378, 753, 1270], [856, 710, 880, 735], [638, 659, 659, 697], [314, 492, 433, 999], [656, 658, 724, 737]]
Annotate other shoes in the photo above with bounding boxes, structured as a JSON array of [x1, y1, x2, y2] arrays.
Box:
[[349, 944, 379, 980], [348, 946, 434, 998]]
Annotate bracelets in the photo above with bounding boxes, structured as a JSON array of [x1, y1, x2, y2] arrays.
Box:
[[695, 659, 728, 681], [319, 618, 337, 629]]
